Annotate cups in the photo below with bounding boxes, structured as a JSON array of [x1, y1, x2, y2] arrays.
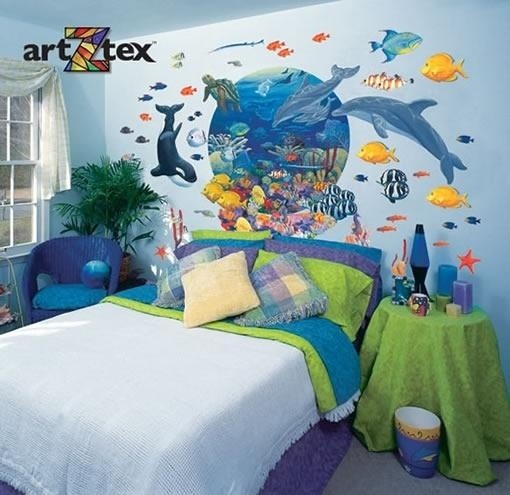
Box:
[[446, 304, 461, 318], [436, 265, 458, 296], [395, 276, 410, 302], [453, 280, 473, 314], [410, 294, 428, 317], [393, 407, 441, 479], [436, 294, 452, 311]]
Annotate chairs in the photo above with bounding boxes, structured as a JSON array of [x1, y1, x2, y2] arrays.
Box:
[[20, 236, 124, 322]]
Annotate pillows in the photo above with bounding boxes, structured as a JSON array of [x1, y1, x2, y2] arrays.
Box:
[[159, 222, 383, 346]]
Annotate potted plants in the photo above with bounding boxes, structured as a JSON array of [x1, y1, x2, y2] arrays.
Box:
[[53, 160, 163, 285]]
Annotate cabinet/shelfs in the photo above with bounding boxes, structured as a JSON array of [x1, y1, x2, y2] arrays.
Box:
[[0, 254, 24, 328]]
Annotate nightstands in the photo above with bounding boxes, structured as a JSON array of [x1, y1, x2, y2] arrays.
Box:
[[355, 297, 510, 486]]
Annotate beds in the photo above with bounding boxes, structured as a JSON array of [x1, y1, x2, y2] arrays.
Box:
[[0, 229, 383, 495]]
